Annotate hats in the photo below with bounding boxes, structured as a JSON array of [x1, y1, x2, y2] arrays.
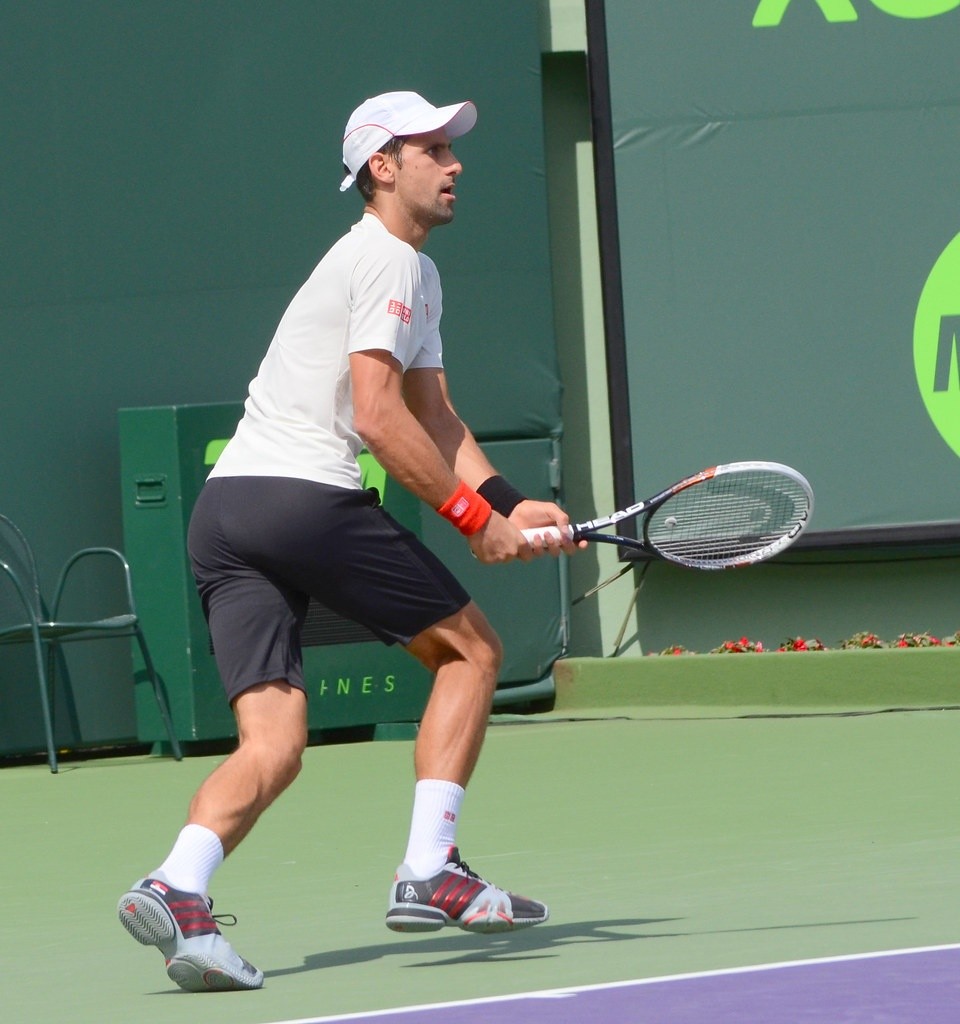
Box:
[[338, 91, 478, 192]]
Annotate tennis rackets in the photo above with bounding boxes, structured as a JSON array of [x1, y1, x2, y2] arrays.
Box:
[[470, 461, 816, 571]]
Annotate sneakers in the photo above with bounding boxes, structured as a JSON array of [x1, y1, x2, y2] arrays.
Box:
[[117, 870, 265, 990], [385, 845, 549, 934]]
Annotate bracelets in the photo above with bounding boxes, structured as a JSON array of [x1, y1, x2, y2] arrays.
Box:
[[476, 474, 528, 517], [435, 478, 492, 535]]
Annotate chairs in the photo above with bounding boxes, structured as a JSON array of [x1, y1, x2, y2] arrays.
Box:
[[0, 513, 184, 774]]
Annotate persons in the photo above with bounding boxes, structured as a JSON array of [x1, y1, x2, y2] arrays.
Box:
[[118, 90, 592, 990]]
